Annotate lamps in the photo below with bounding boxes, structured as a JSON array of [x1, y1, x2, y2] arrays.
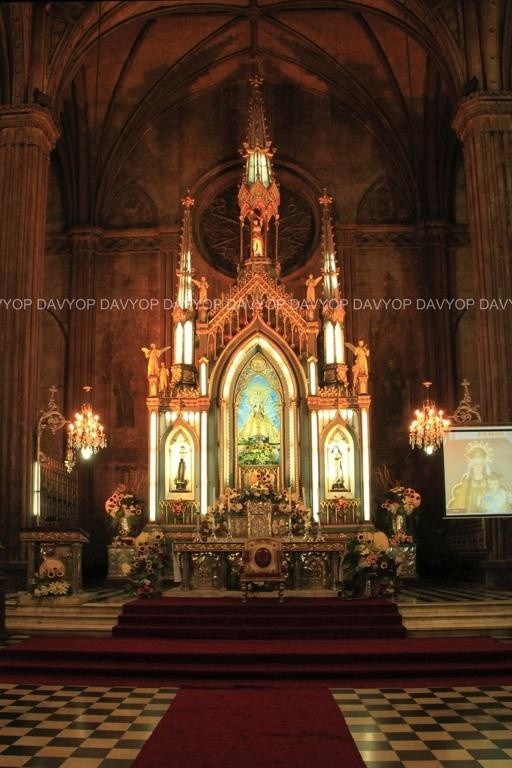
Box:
[[171, 189, 194, 397], [409, 382, 449, 455], [65, 387, 107, 473], [237, 0, 281, 263], [319, 187, 348, 396]]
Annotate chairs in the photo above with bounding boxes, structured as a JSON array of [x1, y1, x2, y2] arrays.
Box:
[[240, 538, 285, 604]]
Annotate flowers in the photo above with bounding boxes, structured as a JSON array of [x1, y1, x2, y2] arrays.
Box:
[[334, 496, 349, 516], [202, 471, 310, 532], [171, 497, 185, 515], [39, 568, 70, 596], [382, 486, 421, 544], [108, 485, 142, 536], [126, 530, 165, 599], [355, 533, 400, 599]]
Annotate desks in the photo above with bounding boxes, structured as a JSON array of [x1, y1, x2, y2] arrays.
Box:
[[172, 540, 346, 591]]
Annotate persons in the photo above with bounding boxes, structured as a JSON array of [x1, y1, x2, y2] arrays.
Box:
[[463, 445, 491, 514], [146, 342, 159, 376], [158, 361, 170, 395], [250, 214, 264, 256], [486, 472, 512, 514], [355, 338, 370, 378], [329, 446, 344, 483]]
